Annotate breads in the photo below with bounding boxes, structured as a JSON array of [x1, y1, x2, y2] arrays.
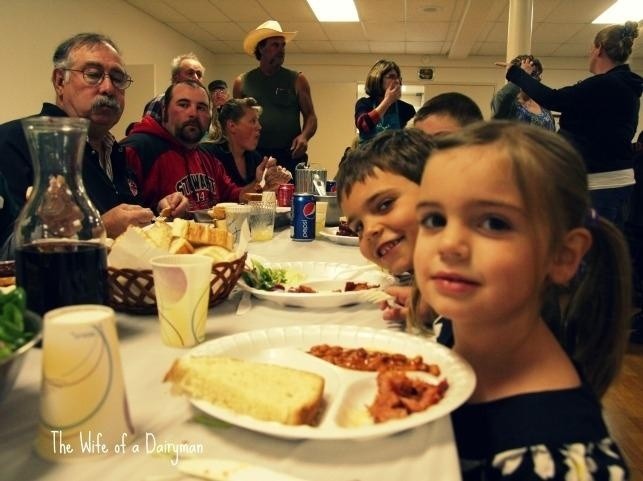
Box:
[[162, 355, 324, 425]]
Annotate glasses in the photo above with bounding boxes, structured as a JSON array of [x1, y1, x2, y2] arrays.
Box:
[[61, 65, 133, 90], [384, 74, 403, 81]]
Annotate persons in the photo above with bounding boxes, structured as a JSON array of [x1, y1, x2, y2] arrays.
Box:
[[491, 54, 557, 132], [119, 82, 254, 215], [198, 98, 277, 186], [333, 130, 440, 325], [356, 60, 416, 136], [414, 93, 485, 138], [412, 122, 631, 481], [1, 32, 190, 270], [209, 80, 231, 111], [143, 56, 206, 120], [495, 21, 643, 343], [235, 19, 318, 175]]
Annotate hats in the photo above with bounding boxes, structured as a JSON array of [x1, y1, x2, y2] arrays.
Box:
[[243, 21, 297, 56]]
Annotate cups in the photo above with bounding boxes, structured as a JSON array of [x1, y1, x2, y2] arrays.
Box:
[[149, 254, 214, 350], [247, 200, 276, 242], [32, 304, 139, 466], [224, 205, 253, 244], [314, 202, 329, 240]]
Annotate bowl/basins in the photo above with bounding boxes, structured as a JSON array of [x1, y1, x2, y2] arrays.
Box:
[[303, 192, 343, 226], [0, 309, 42, 368], [185, 210, 214, 224], [318, 228, 359, 246]]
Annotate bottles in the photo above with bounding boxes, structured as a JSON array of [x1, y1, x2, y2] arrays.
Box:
[[290, 193, 316, 242], [277, 184, 295, 225], [326, 181, 337, 195]]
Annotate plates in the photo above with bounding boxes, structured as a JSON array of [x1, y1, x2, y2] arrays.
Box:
[[174, 325, 477, 442], [236, 262, 399, 309]]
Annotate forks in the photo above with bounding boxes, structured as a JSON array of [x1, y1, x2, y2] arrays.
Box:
[[258, 157, 272, 190]]
